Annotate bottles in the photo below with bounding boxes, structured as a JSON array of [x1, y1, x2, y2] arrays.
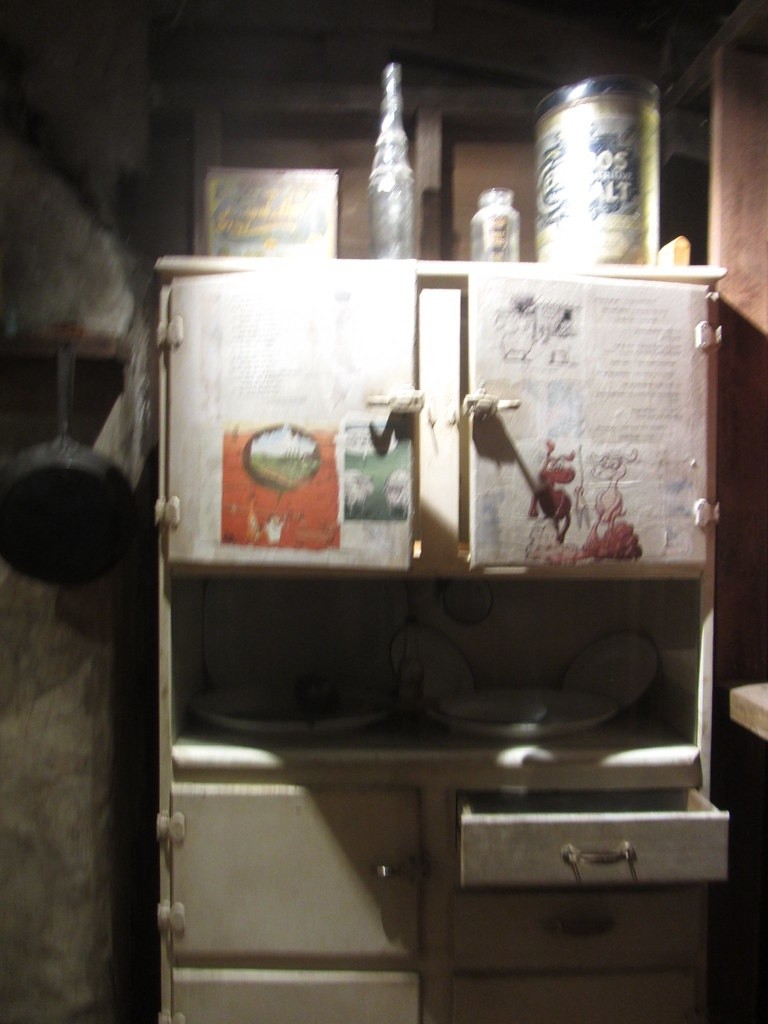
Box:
[[469, 189, 518, 263]]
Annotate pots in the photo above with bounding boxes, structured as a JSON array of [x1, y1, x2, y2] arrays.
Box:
[[0, 312, 137, 588]]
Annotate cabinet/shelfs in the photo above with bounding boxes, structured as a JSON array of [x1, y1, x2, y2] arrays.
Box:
[[150, 253, 731, 1024]]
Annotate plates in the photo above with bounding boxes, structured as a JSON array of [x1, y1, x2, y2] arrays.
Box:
[[425, 688, 618, 739], [565, 633, 660, 712], [190, 679, 402, 733]]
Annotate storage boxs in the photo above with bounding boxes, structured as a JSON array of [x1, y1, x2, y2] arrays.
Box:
[[203, 166, 338, 258]]
